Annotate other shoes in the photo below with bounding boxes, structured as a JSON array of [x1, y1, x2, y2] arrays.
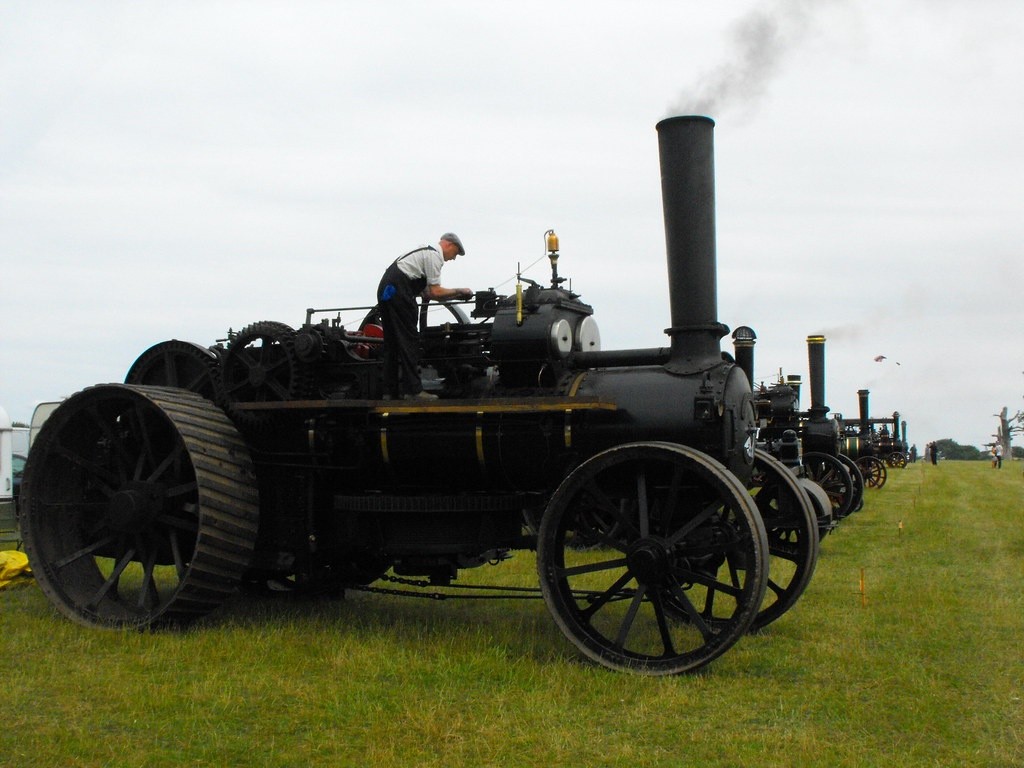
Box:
[[405, 390, 439, 401]]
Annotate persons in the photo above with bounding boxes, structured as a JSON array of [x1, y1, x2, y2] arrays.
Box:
[[377, 232, 473, 400], [925, 441, 938, 466], [910, 444, 917, 463], [990, 441, 1003, 469], [880, 425, 889, 439]]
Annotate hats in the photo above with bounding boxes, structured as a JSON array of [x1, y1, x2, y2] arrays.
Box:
[[441, 233, 465, 256]]
